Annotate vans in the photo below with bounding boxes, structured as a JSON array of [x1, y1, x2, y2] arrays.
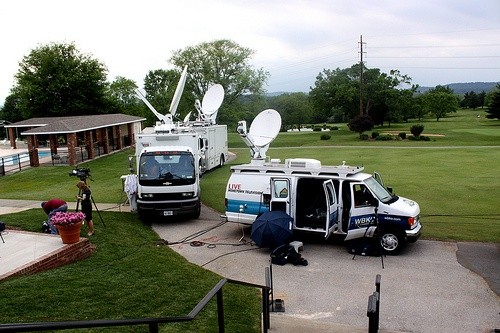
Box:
[[219, 109, 423, 256]]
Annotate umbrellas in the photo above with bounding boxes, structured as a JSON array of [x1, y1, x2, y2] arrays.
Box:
[[251, 210, 294, 249]]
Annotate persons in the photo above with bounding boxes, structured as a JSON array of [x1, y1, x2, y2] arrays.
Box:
[[357, 184, 366, 193], [75, 181, 95, 237], [41, 199, 67, 234]]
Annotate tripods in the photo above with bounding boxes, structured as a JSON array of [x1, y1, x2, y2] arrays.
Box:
[[76, 178, 106, 227], [352, 209, 385, 269]]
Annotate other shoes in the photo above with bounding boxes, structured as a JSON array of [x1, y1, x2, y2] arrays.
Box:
[[88, 231, 94, 237]]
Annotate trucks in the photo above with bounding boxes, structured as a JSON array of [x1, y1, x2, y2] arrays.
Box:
[[128, 65, 229, 226]]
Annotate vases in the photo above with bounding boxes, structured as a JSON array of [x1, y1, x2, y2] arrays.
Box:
[[54, 222, 84, 244]]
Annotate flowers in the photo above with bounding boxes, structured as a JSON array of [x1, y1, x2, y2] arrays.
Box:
[[50, 211, 88, 224]]
